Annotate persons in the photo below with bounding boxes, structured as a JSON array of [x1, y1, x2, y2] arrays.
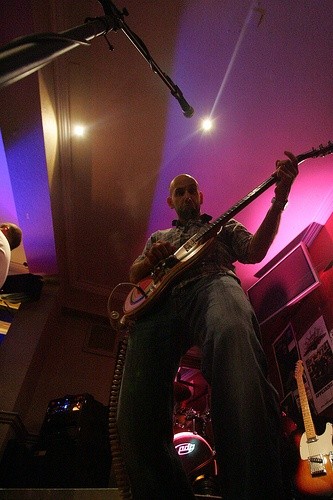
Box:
[[0, 222, 22, 289], [118, 150, 300, 500]]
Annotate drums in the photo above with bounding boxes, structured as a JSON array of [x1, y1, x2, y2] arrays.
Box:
[[173, 409, 207, 437], [173, 432, 217, 487]]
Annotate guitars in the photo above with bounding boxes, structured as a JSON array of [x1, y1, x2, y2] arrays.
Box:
[[121, 141, 333, 318], [294, 360, 333, 493]]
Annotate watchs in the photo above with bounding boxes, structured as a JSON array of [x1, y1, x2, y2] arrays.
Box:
[[271, 197, 289, 210]]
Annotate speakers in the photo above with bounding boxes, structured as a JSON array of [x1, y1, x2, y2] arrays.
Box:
[[0, 273, 44, 303]]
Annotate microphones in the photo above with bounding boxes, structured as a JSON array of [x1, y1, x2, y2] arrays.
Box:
[[171, 87, 194, 118]]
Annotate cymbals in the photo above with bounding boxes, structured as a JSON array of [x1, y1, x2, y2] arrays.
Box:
[[170, 381, 192, 402]]
